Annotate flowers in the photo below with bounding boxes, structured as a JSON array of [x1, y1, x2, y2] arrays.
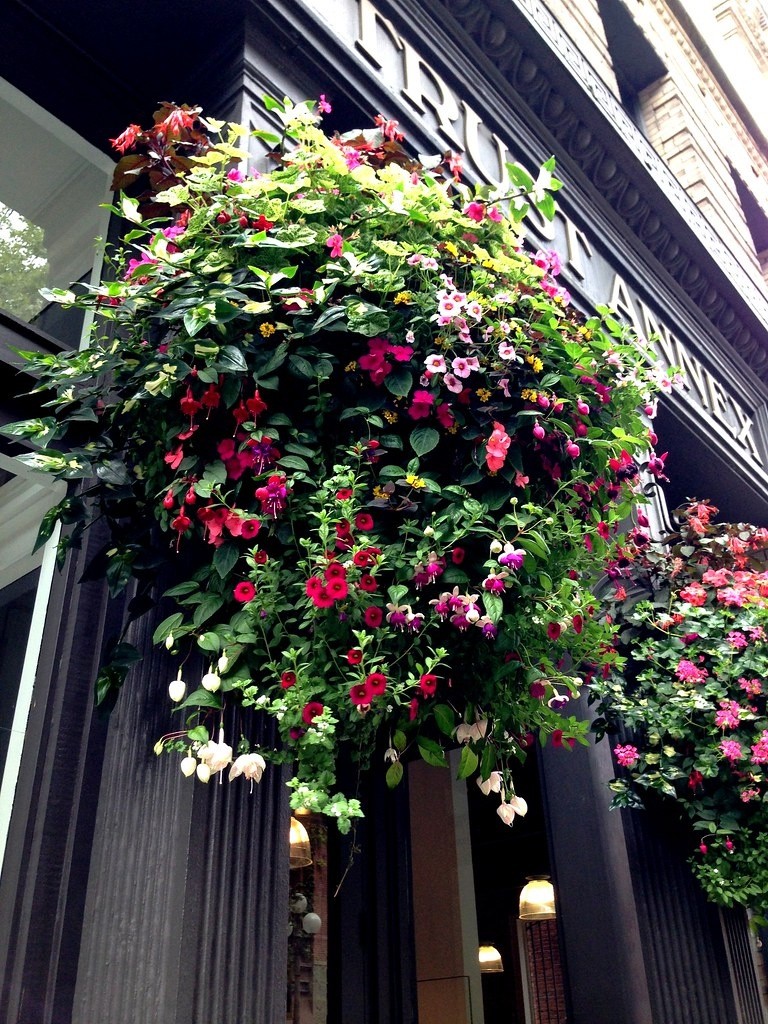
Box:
[[0, 90, 768, 911]]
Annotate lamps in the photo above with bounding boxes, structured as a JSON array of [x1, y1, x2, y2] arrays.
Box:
[[479, 940, 507, 976], [518, 869, 560, 924], [289, 811, 318, 870]]
